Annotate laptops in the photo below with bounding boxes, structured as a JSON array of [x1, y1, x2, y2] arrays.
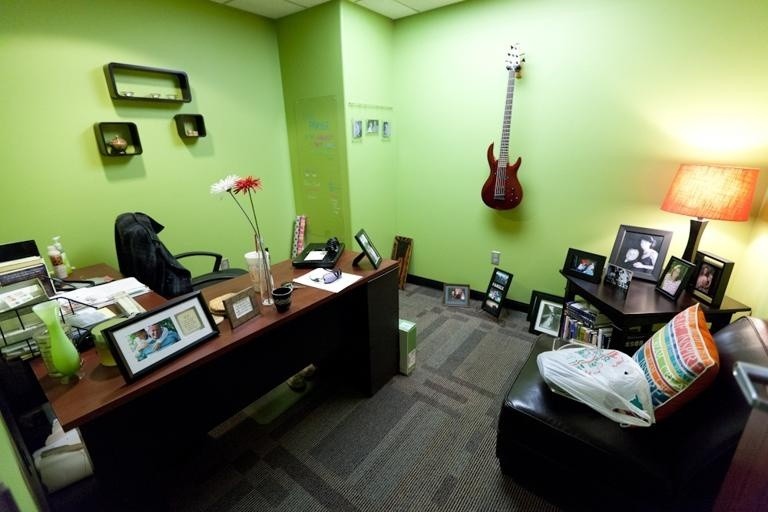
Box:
[[291, 243, 345, 270]]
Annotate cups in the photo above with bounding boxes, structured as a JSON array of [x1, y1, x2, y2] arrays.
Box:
[[168, 94, 177, 100], [244, 251, 275, 293], [151, 93, 160, 98], [124, 92, 134, 97], [272, 287, 292, 314]]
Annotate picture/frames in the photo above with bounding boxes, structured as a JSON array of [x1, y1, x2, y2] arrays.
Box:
[[527, 292, 564, 338], [442, 282, 471, 309], [222, 286, 261, 329], [389, 235, 414, 290], [562, 224, 735, 310], [98, 289, 221, 385], [524, 290, 542, 322], [350, 228, 383, 271], [481, 267, 514, 319]]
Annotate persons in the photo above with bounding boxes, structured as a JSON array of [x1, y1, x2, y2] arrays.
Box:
[[659, 265, 683, 296], [136, 326, 168, 352], [695, 265, 713, 290], [624, 235, 659, 274], [613, 268, 631, 290], [138, 324, 179, 361]]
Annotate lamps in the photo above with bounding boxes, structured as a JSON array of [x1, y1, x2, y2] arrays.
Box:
[[657, 161, 759, 287]]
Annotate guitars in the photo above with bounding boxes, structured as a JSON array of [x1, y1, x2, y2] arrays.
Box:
[[481, 42, 523, 210]]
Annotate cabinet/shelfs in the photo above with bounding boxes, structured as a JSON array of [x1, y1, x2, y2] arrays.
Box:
[[92, 59, 208, 160]]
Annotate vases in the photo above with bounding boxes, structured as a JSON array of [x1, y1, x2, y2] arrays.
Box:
[[31, 298, 84, 388], [254, 236, 277, 305]]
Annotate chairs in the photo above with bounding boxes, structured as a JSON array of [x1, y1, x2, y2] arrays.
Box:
[[492, 315, 767, 512], [113, 211, 251, 300]]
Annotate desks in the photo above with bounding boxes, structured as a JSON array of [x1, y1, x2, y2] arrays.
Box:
[[558, 269, 751, 359], [25, 250, 403, 512]]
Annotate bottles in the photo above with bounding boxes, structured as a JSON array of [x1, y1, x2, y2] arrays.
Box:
[[47, 245, 69, 279], [32, 299, 85, 386], [110, 135, 128, 155], [89, 317, 133, 368]]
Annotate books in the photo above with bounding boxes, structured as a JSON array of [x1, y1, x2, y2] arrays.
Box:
[[0, 256, 151, 361], [563, 300, 656, 357]]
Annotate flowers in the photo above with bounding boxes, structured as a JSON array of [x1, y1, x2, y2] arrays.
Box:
[[208, 173, 274, 304]]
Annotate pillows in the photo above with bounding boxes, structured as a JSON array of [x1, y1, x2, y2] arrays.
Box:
[[615, 301, 721, 429]]
